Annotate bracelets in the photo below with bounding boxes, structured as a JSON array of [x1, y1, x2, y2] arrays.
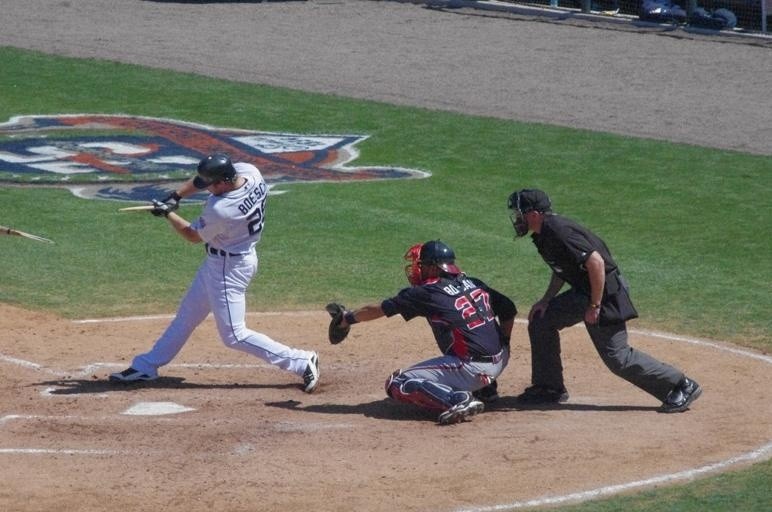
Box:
[[587, 301, 601, 309]]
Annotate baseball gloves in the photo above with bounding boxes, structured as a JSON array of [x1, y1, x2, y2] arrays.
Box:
[[325, 302, 350, 345]]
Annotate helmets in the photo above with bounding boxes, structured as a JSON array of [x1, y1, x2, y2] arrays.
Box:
[[404, 239, 461, 286], [507, 189, 558, 237], [193, 153, 236, 189]]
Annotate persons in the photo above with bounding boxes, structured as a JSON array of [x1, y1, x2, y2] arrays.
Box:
[[505, 187, 703, 414], [324, 238, 519, 426], [109, 150, 322, 394]]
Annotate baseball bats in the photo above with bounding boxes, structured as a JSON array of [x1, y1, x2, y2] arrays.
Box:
[[119, 205, 155, 212]]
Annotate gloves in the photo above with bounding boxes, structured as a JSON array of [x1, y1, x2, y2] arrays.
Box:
[[150, 191, 182, 218]]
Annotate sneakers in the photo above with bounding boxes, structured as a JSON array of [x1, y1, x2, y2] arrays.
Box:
[[109, 368, 159, 382], [302, 350, 320, 393], [656, 376, 701, 413], [518, 385, 568, 405], [438, 390, 485, 425], [472, 380, 499, 402]]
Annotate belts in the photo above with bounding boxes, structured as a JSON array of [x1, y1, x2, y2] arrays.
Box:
[[472, 355, 493, 363], [204, 243, 243, 256]]
